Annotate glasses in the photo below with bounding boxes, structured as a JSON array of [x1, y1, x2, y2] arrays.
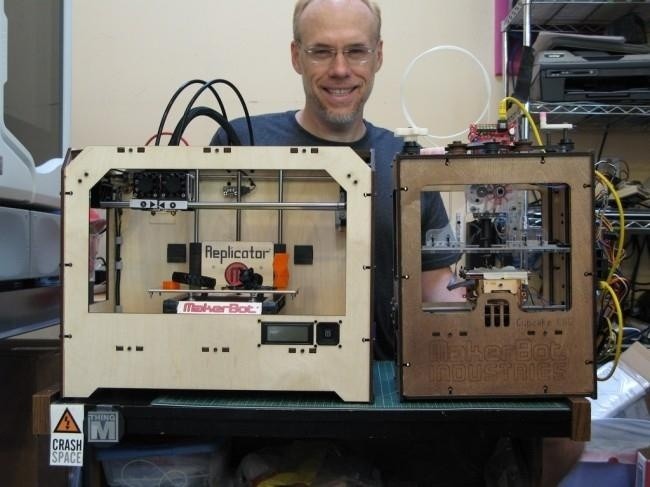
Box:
[[295, 39, 380, 65]]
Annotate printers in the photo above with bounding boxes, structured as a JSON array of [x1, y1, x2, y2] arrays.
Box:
[[530, 47, 650, 106]]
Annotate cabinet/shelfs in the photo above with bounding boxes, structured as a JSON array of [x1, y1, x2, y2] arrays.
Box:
[[500, 1, 649, 368]]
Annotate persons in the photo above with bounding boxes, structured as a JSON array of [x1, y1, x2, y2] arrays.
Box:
[[208, 0, 468, 362]]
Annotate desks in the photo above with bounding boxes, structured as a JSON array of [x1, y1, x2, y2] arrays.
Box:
[[6, 323, 61, 385], [30, 382, 591, 487]]
